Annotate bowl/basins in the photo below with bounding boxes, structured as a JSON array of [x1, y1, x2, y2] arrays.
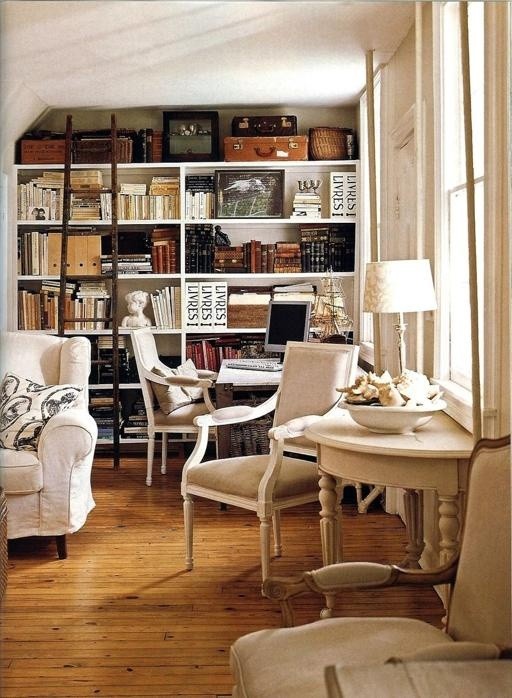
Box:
[[337, 399, 447, 435]]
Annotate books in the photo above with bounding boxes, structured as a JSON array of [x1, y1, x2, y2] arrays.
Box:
[[185, 334, 280, 372], [18, 279, 113, 330], [100, 224, 180, 276], [89, 396, 125, 439], [330, 171, 356, 218], [17, 170, 180, 220], [289, 193, 321, 219], [98, 335, 129, 383], [185, 175, 215, 219], [122, 390, 150, 439], [150, 286, 180, 328], [185, 223, 355, 274], [185, 281, 228, 329], [16, 231, 49, 276]]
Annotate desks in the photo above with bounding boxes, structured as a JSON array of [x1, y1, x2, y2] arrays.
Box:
[[303, 414, 474, 635], [214, 358, 375, 511]]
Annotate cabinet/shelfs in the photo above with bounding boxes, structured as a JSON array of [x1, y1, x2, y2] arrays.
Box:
[[12, 161, 365, 443]]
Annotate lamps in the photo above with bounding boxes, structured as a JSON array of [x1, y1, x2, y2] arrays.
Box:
[[362, 258, 438, 372]]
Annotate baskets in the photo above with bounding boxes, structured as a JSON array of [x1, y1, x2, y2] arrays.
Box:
[[68, 137, 134, 164], [312, 128, 353, 160]]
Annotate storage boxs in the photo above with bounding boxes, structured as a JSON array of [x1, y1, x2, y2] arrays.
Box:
[[73, 139, 132, 163], [20, 140, 67, 164], [227, 306, 268, 328]]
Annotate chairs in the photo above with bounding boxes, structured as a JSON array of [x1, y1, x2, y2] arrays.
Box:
[[0, 331, 98, 559], [228, 432, 511, 698], [179, 340, 358, 594]]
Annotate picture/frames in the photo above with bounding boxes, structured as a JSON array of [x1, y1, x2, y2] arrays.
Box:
[[162, 110, 219, 161], [215, 171, 285, 220]]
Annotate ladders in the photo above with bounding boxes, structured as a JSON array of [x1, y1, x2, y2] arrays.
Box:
[[57, 111, 119, 471]]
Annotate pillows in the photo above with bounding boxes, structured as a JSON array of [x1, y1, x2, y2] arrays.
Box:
[[0, 371, 84, 452], [150, 359, 207, 413]]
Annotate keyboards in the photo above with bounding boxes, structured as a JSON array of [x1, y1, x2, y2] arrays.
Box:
[[227, 361, 285, 372]]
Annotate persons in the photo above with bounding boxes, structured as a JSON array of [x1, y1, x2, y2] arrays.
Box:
[[121, 290, 151, 328]]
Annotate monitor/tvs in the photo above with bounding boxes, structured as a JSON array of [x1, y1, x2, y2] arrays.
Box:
[[265, 302, 312, 354]]
